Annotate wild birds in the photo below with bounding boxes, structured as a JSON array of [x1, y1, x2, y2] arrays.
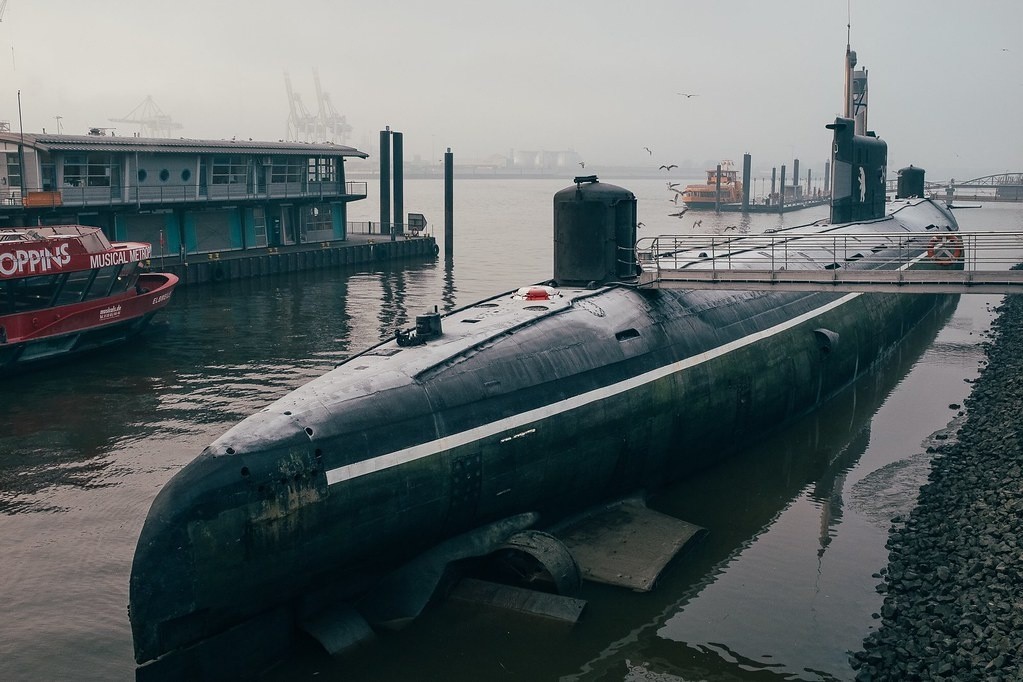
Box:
[[666, 181, 693, 197], [724, 226, 738, 232], [659, 165, 678, 172], [669, 194, 678, 205], [636, 222, 645, 229], [677, 92, 700, 98], [643, 147, 652, 155], [692, 219, 703, 229], [668, 207, 688, 219], [579, 161, 586, 168]]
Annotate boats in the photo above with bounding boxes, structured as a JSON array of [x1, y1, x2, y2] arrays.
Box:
[[0, 223, 181, 372], [681, 160, 744, 208], [125, 0, 968, 666]]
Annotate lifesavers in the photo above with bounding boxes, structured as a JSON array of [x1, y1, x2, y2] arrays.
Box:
[[412, 227, 419, 236], [927, 233, 962, 266], [431, 243, 439, 255]]
